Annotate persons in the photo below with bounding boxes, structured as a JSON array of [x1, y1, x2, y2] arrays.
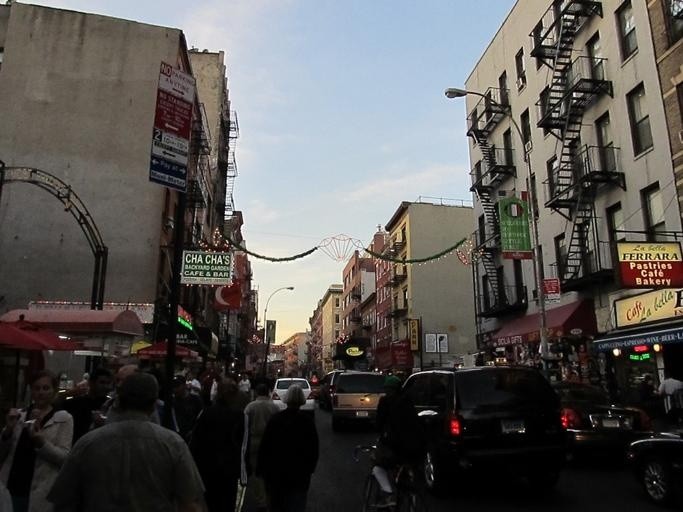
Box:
[[640, 374, 655, 398], [1, 365, 327, 512], [518, 344, 586, 383], [653, 373, 683, 428], [370, 374, 421, 507]]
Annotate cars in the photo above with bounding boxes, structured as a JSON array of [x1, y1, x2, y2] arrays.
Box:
[[265, 377, 316, 417]]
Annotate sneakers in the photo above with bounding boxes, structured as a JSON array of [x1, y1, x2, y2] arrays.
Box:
[[368, 494, 397, 508]]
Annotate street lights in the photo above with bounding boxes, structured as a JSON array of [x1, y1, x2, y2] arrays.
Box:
[[442, 89, 549, 377], [262, 284, 296, 349]]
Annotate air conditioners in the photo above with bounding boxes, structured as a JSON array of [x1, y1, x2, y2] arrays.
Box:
[[524, 141, 532, 152], [515, 77, 524, 90]]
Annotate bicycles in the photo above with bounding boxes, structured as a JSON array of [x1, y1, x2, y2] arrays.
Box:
[[352, 436, 427, 512]]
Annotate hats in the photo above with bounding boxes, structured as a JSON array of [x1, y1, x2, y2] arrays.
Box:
[[383, 375, 401, 388], [170, 375, 202, 391], [284, 385, 306, 405]]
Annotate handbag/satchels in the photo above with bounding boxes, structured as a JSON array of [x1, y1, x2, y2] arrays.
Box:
[[181, 430, 193, 447]]
